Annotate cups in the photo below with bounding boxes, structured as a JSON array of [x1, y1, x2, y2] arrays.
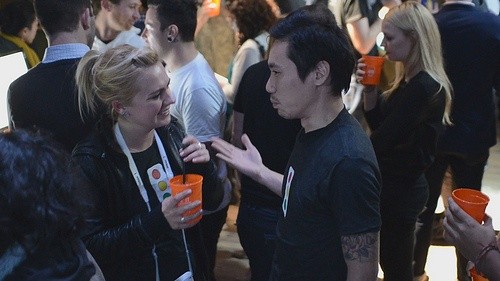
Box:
[[205, 0, 220, 17], [169, 174, 203, 223], [362, 55, 385, 85], [452, 189, 490, 224]]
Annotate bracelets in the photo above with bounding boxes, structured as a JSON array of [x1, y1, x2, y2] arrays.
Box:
[[475, 243, 500, 272]]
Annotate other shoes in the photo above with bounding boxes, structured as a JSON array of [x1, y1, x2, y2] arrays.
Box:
[[431, 212, 446, 246]]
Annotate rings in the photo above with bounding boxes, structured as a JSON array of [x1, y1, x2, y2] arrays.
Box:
[[181, 217, 184, 222]]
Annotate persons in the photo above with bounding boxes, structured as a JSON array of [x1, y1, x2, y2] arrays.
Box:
[[230, 61, 302, 281], [431, 0, 500, 193], [91, 0, 144, 52], [223, 0, 279, 100], [442, 198, 500, 281], [68, 44, 225, 281], [0, 0, 41, 68], [211, 8, 382, 281], [0, 128, 105, 281], [355, 0, 452, 281], [327, 0, 403, 121], [7, 0, 109, 151], [141, 0, 232, 281]]
